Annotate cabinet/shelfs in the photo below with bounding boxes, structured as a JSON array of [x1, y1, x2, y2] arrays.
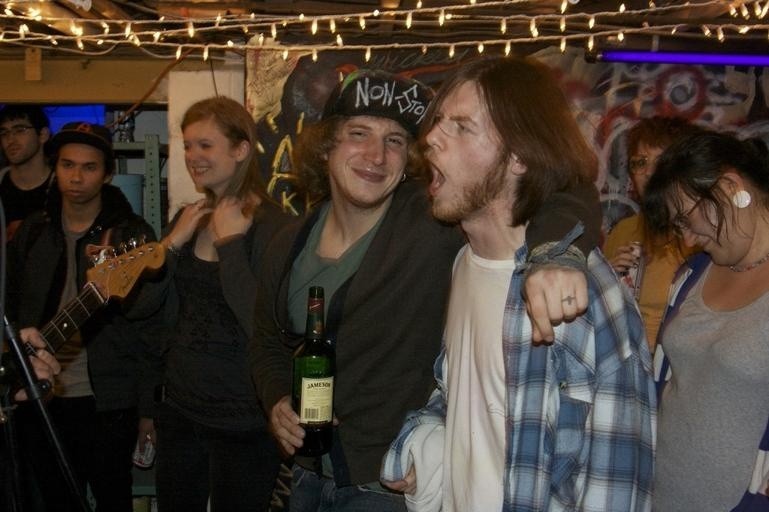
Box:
[[84, 135, 169, 512]]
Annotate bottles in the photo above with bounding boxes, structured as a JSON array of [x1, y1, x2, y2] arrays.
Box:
[[288, 286, 338, 458]]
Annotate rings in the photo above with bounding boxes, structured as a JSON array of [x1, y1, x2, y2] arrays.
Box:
[[558, 295, 578, 307], [195, 203, 201, 208]]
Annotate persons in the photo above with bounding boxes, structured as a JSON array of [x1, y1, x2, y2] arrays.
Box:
[[7, 324, 64, 402], [603, 117, 719, 355], [650, 130, 769, 512], [247, 66, 588, 510], [0, 105, 59, 244], [129, 97, 294, 511], [2, 121, 171, 512], [378, 58, 657, 512]]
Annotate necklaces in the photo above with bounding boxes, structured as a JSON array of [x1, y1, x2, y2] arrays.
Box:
[[729, 249, 769, 271]]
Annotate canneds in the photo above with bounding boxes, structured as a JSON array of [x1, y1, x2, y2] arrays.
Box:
[[132, 434, 158, 468], [620, 241, 646, 302]]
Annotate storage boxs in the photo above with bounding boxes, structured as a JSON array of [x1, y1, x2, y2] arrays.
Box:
[[46, 103, 106, 137]]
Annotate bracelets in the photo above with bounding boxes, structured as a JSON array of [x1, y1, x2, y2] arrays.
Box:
[[166, 234, 181, 257]]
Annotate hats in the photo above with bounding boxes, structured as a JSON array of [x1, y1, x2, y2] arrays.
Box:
[[322, 68, 438, 139], [41, 121, 117, 173]]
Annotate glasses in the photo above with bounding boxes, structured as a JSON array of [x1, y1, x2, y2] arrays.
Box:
[[674, 172, 724, 232], [0, 125, 39, 141], [626, 151, 665, 178]]
